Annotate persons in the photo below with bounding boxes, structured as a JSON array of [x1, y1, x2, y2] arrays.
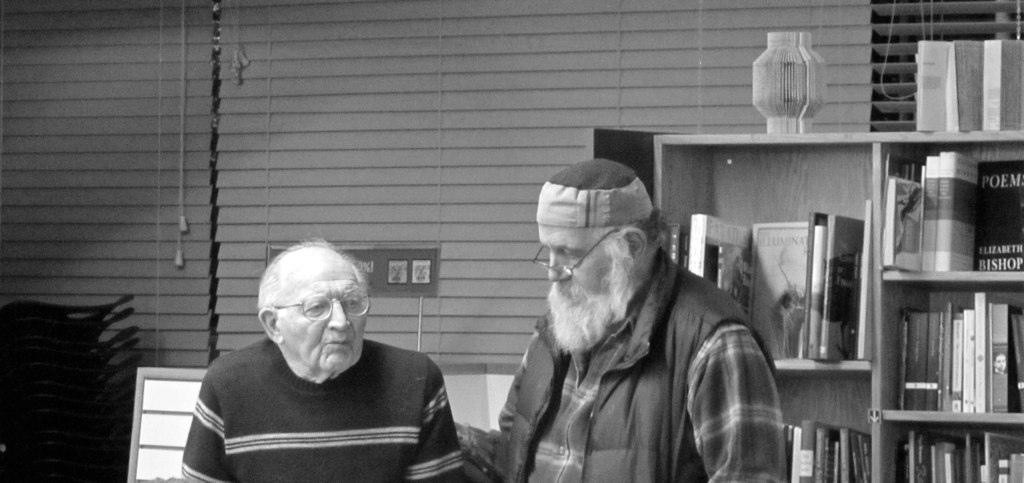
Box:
[[452, 156, 790, 482], [176, 238, 463, 481], [993, 353, 1006, 375]]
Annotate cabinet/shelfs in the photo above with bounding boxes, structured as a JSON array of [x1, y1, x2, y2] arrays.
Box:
[[651, 128, 1024, 483]]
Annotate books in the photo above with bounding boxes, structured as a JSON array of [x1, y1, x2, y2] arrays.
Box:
[[972, 161, 1022, 272], [914, 38, 1024, 135], [897, 292, 1023, 412], [666, 200, 874, 361], [880, 151, 979, 272], [784, 420, 871, 482], [894, 432, 1023, 483]]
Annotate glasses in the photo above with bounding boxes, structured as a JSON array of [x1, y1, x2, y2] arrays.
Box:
[[527, 228, 622, 279], [271, 287, 372, 322]]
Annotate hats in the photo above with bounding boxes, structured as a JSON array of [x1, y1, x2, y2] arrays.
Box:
[[537, 159, 654, 229]]
